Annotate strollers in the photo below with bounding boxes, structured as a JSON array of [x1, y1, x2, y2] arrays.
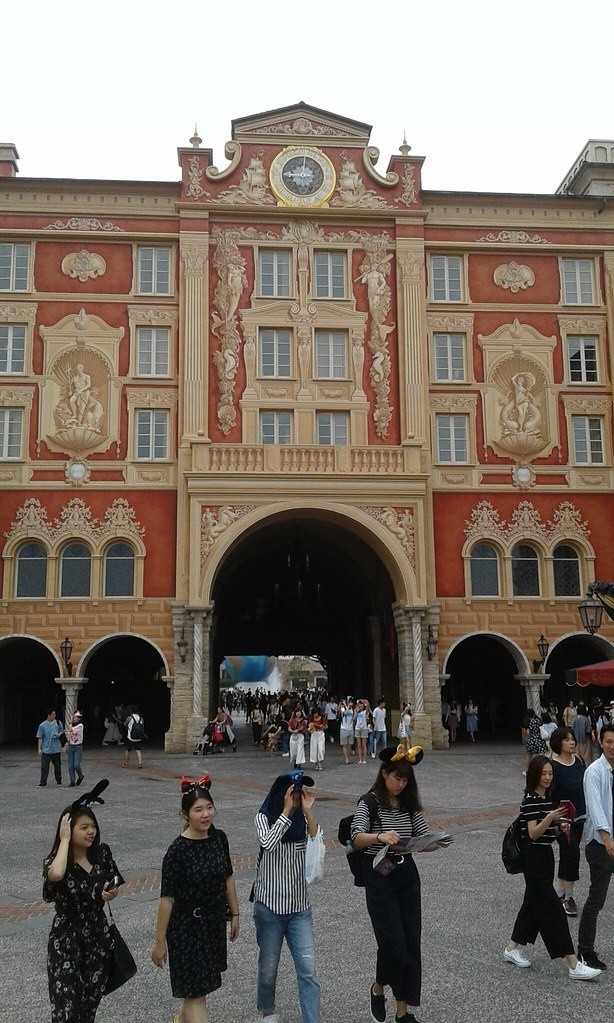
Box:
[[193, 721, 224, 755]]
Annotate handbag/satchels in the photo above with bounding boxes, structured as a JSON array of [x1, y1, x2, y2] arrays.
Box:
[[57, 721, 67, 748], [536, 716, 552, 740], [102, 897, 137, 996], [305, 822, 325, 884]]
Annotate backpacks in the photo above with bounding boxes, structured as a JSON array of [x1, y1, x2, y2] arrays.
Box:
[[338, 792, 380, 885], [105, 718, 110, 728], [131, 716, 143, 739], [501, 816, 524, 874]]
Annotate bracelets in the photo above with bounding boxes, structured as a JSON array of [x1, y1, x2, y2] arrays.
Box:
[[231, 914, 240, 916], [377, 832, 382, 842]]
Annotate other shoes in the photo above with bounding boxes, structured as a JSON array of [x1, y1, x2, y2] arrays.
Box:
[[56, 778, 61, 786], [36, 783, 47, 788], [577, 952, 607, 970], [118, 742, 124, 745], [282, 752, 375, 771], [102, 743, 108, 745], [261, 1013, 277, 1023], [523, 771, 526, 776], [69, 782, 75, 787], [173, 1015, 180, 1023], [76, 775, 84, 786]]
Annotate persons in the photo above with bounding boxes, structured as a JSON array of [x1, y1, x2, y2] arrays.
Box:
[[42, 804, 126, 1023], [503, 755, 601, 980], [151, 773, 240, 1023], [576, 724, 614, 971], [249, 770, 323, 1023], [350, 746, 429, 1023], [67, 264, 539, 432], [35, 686, 614, 791], [544, 727, 587, 916]]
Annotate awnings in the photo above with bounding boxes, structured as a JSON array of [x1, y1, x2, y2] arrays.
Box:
[[565, 658, 614, 688]]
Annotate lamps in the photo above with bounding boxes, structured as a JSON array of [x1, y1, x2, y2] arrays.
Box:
[[59, 637, 74, 677], [425, 624, 439, 661], [532, 634, 550, 671], [177, 626, 189, 663]]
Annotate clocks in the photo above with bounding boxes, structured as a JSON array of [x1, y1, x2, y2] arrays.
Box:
[[269, 146, 337, 205]]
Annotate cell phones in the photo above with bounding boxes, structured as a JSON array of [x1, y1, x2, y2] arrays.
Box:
[[560, 799, 576, 821], [106, 875, 119, 892]]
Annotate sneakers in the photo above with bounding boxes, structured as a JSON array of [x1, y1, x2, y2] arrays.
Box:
[[369, 981, 387, 1023], [561, 894, 578, 915], [394, 1012, 419, 1023], [504, 947, 532, 967], [568, 954, 601, 979]]
